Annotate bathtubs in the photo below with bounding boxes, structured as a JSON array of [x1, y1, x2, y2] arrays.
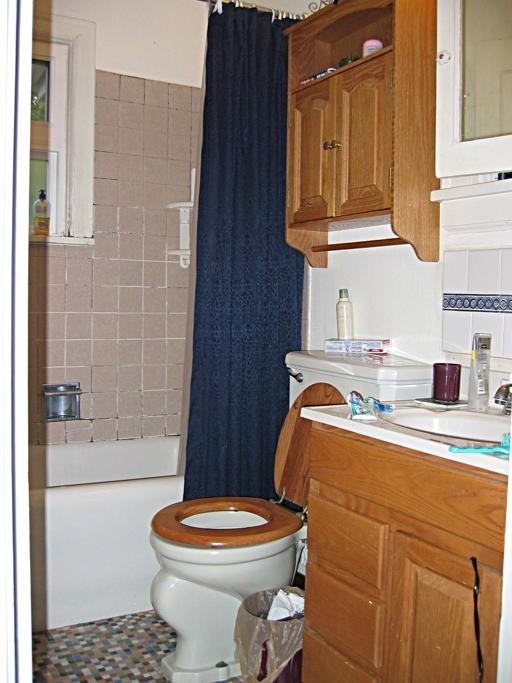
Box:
[[28, 430, 185, 631]]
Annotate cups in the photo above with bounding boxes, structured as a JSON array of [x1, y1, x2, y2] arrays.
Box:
[[432, 363, 462, 403]]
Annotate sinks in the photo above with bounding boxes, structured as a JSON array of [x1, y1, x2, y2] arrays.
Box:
[[381, 408, 512, 442]]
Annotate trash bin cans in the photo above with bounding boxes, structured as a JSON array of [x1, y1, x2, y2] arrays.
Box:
[[240, 586, 305, 683]]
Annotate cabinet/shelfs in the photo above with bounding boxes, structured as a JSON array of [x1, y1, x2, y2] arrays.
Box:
[[301, 421, 508, 683], [283, 0, 440, 269]]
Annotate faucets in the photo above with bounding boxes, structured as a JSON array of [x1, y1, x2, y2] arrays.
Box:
[[493, 384, 511, 405]]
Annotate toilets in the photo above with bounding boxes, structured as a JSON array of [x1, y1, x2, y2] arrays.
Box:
[[150, 344, 432, 683]]
